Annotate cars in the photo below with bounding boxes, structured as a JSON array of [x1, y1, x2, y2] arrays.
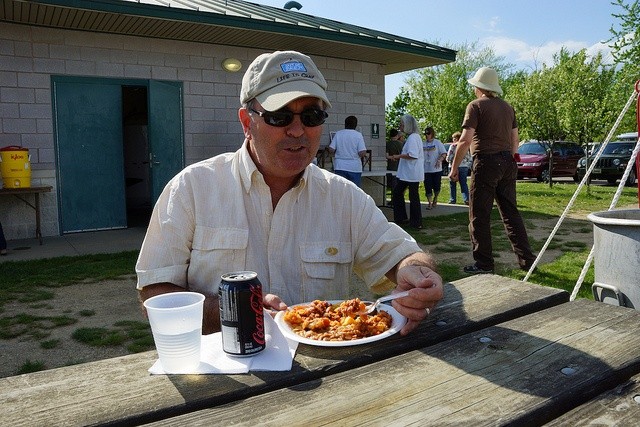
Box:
[[442, 143, 451, 176]]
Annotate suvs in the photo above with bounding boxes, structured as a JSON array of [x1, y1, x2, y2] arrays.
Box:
[[578, 141, 638, 188]]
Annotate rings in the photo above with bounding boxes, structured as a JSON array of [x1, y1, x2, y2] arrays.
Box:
[[424, 306, 433, 324]]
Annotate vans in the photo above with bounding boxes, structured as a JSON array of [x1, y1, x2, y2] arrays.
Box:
[[516, 140, 585, 182]]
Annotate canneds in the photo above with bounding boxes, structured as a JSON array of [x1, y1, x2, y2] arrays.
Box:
[[218, 270, 266, 359]]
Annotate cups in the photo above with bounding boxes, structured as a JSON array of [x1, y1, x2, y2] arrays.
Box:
[[143, 292, 206, 372]]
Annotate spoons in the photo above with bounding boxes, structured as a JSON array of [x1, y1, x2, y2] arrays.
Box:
[[354, 285, 436, 314]]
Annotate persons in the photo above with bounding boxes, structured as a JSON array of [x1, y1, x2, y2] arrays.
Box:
[[136, 47, 445, 337], [447, 66, 539, 274], [385, 128, 403, 208], [422, 125, 447, 209], [390, 111, 425, 232], [328, 109, 365, 195], [446, 130, 471, 207]]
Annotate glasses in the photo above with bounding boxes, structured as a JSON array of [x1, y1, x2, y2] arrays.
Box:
[[246, 101, 330, 129], [423, 132, 430, 135]]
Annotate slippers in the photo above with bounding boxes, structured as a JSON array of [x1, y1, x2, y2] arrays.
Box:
[[426, 206, 433, 210]]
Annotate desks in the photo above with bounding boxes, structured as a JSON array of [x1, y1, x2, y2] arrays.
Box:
[[361, 166, 401, 209], [1, 184, 52, 249], [0, 271, 639, 425]]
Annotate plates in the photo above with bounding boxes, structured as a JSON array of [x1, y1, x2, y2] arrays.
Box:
[[274, 299, 407, 347]]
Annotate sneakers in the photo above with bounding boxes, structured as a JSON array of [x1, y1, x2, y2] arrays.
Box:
[[448, 200, 456, 204], [464, 264, 494, 275]]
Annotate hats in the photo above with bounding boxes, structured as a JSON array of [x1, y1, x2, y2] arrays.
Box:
[[390, 129, 398, 137], [238, 50, 334, 111], [466, 65, 504, 94]]
